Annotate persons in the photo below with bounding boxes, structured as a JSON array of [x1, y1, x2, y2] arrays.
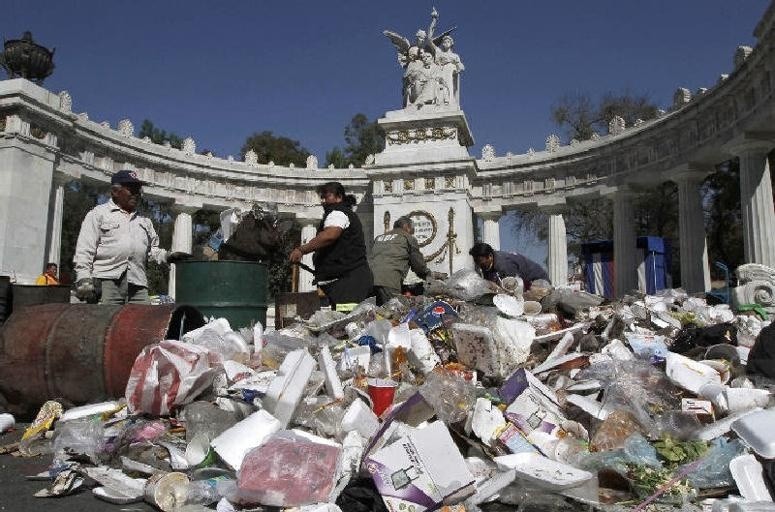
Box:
[[289, 181, 374, 310], [76, 170, 192, 305], [398, 6, 466, 110], [36, 262, 60, 286], [469, 244, 551, 290], [368, 216, 448, 309]]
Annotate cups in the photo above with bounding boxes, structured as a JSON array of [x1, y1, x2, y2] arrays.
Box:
[[185, 434, 216, 469], [367, 377, 397, 417]]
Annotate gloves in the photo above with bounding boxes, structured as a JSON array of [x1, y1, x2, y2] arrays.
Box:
[[74, 277, 98, 303], [166, 249, 195, 263]]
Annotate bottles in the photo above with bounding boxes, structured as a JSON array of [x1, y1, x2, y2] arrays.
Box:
[[163, 475, 230, 508]]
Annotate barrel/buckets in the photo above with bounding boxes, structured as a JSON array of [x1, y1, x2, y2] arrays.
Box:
[[0, 302, 206, 421], [10, 285, 71, 317], [174, 261, 267, 334], [0, 276, 10, 329], [275, 292, 319, 330]]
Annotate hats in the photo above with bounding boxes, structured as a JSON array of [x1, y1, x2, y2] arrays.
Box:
[[110, 169, 143, 185]]
